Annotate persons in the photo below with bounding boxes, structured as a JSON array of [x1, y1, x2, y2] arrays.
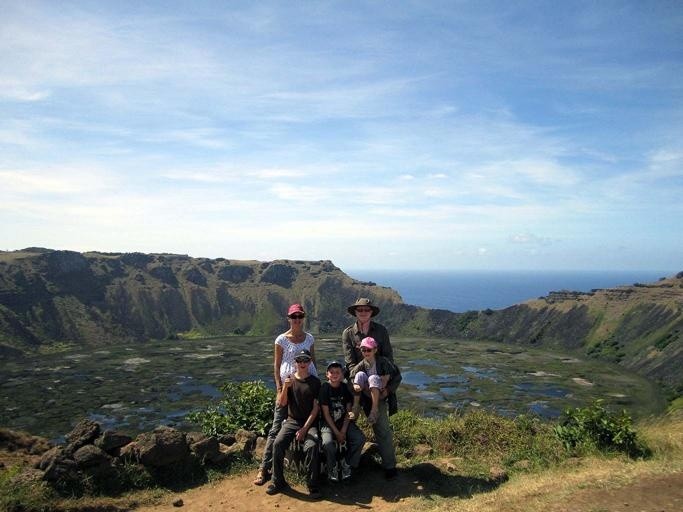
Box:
[[345, 334, 401, 426], [316, 359, 365, 487], [253, 303, 318, 485], [266, 349, 323, 500], [341, 297, 401, 481]]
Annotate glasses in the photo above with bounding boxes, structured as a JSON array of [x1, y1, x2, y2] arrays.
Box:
[[355, 309, 372, 312], [287, 314, 304, 319], [294, 357, 310, 363], [361, 348, 372, 352]]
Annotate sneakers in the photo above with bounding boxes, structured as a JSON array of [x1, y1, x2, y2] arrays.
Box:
[[383, 468, 398, 481], [360, 441, 379, 456], [266, 481, 289, 495], [366, 409, 380, 424], [347, 404, 360, 420], [254, 471, 272, 486]]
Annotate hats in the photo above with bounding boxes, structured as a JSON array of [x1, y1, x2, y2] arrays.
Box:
[[327, 360, 342, 372], [360, 336, 378, 349], [288, 304, 305, 315], [293, 349, 312, 358], [348, 298, 380, 317]]
